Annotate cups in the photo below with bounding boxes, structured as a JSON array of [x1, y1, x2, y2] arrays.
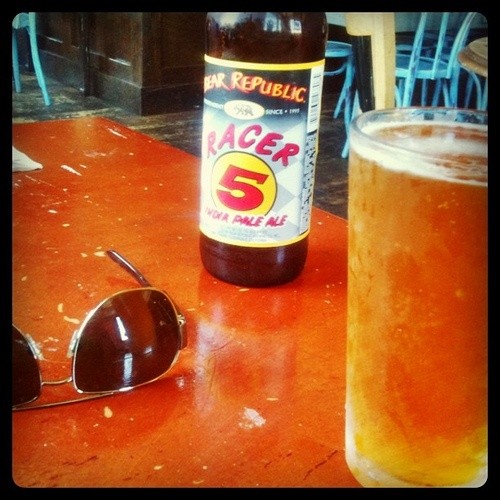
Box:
[[344, 104, 488, 488]]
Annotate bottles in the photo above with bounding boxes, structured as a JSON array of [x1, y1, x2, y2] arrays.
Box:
[[199, 11, 327, 288]]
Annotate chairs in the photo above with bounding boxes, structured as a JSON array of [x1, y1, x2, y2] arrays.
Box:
[[11, 11, 53, 106], [344, 11, 400, 116], [322, 36, 380, 159], [391, 12, 476, 112]]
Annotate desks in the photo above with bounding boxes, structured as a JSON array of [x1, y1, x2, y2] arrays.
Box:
[[457, 36, 488, 84]]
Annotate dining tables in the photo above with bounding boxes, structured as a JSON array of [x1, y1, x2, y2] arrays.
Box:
[[13, 114, 375, 488]]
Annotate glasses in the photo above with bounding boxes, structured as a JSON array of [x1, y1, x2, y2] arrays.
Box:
[[13, 250, 187, 411]]
